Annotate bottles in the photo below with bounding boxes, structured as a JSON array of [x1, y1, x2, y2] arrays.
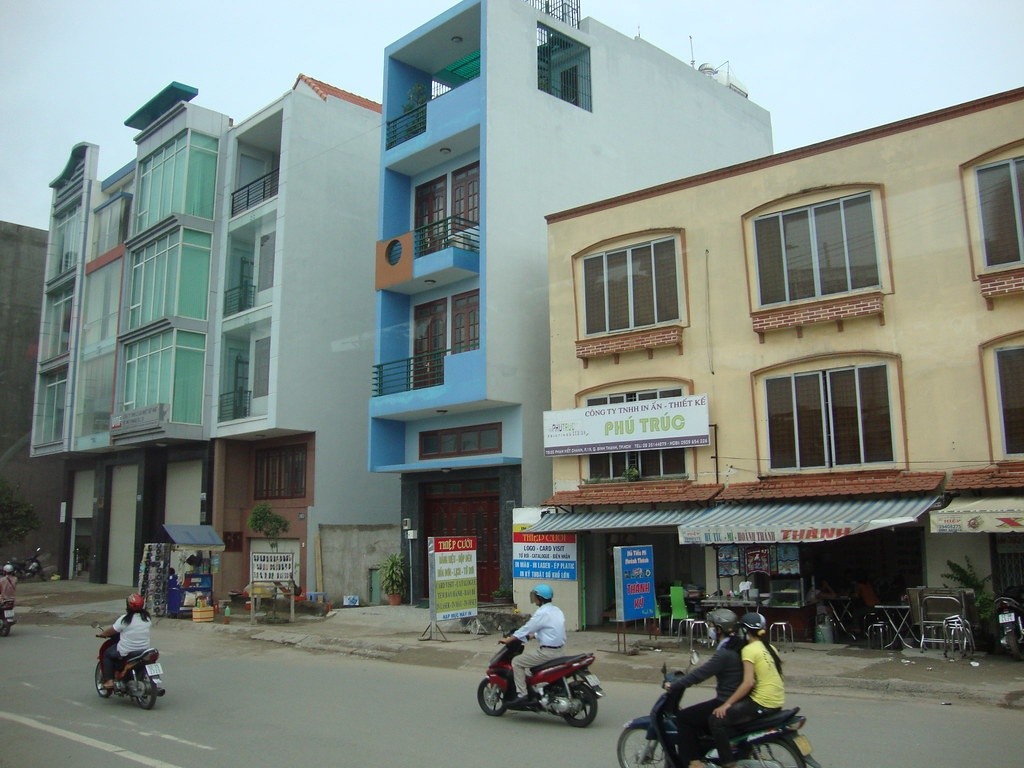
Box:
[[197, 595, 207, 608], [727, 592, 730, 599], [225, 606, 230, 616], [200, 558, 209, 574]]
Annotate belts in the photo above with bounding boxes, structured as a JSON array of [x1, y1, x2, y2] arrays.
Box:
[[540, 645, 563, 649]]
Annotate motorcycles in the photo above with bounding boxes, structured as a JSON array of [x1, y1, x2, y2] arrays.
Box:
[[90, 620, 166, 710], [0, 597, 19, 636], [6, 546, 48, 583], [617, 650, 822, 768], [993, 585, 1024, 662], [477, 628, 605, 728]]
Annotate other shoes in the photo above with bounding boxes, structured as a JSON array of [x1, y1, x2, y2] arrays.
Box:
[[722, 762, 739, 768], [104, 681, 114, 687], [507, 694, 529, 706], [688, 760, 706, 768]]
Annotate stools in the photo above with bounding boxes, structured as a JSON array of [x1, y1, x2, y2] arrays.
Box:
[[943, 614, 976, 659], [307, 591, 327, 604], [644, 613, 715, 651], [769, 622, 795, 653], [864, 613, 893, 650]]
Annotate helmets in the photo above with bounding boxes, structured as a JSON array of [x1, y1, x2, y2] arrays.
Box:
[[127, 593, 144, 610], [3, 564, 13, 572], [740, 612, 766, 629], [530, 584, 553, 599], [706, 609, 737, 635]]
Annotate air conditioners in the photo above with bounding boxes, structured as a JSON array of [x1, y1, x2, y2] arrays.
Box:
[[448, 233, 471, 250]]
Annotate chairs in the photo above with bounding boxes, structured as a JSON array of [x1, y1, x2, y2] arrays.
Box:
[[654, 587, 696, 635]]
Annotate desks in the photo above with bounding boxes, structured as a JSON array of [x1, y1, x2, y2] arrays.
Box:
[[824, 596, 857, 641], [906, 586, 975, 654], [874, 604, 920, 648]]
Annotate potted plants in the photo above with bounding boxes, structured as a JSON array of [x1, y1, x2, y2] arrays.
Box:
[[492, 576, 513, 604], [943, 557, 1001, 655], [376, 552, 406, 605]]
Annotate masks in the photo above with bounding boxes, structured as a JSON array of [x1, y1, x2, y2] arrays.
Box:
[[708, 626, 718, 643]]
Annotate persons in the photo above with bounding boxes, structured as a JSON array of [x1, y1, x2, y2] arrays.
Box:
[[168, 567, 175, 577], [847, 568, 889, 633], [288, 580, 298, 596], [0, 564, 18, 621], [96, 593, 153, 688], [5, 558, 42, 578], [712, 611, 786, 767], [664, 608, 748, 768], [497, 583, 567, 707], [802, 558, 823, 600]]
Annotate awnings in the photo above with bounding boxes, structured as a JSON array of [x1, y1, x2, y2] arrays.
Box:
[[521, 506, 714, 534], [677, 492, 944, 545], [928, 497, 1024, 534]]
